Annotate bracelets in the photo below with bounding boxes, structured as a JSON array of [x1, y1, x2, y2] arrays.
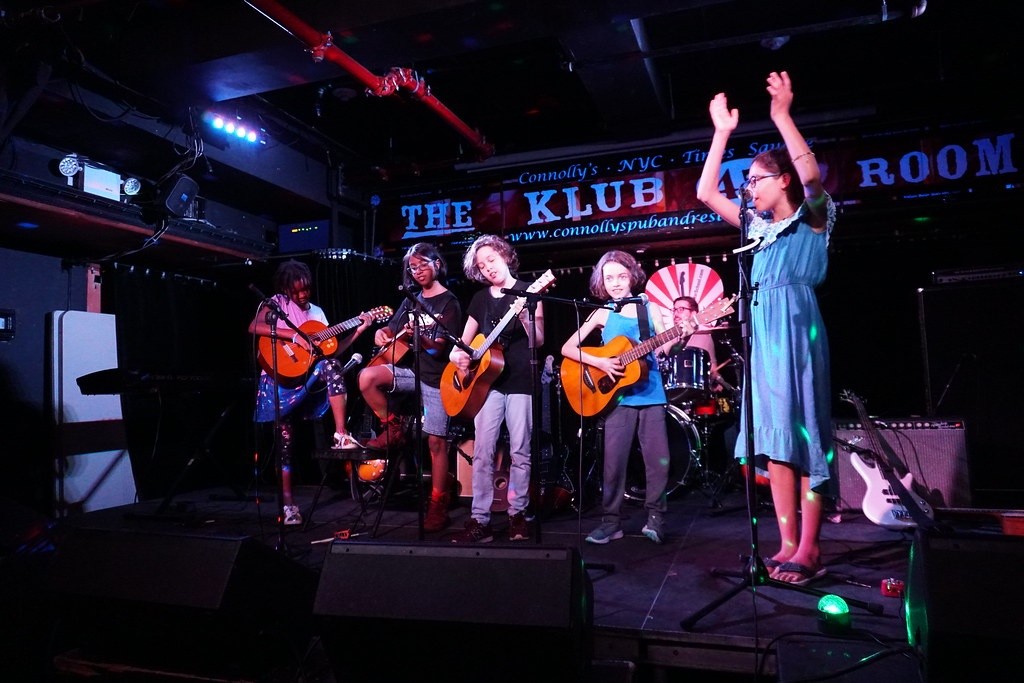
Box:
[[293, 333, 299, 343], [792, 153, 815, 163]]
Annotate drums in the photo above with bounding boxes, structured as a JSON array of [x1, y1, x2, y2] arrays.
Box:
[[665, 345, 711, 401], [596, 404, 701, 502]]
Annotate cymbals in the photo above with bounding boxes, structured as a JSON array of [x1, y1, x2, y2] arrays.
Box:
[[697, 324, 740, 334]]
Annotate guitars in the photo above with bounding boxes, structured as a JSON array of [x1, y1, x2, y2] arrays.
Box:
[[256, 305, 394, 388], [527, 355, 578, 513], [558, 292, 738, 419], [364, 326, 414, 367], [440, 268, 558, 421], [838, 388, 935, 530], [345, 343, 390, 484]]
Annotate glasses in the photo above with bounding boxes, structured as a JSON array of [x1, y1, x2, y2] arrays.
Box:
[[407, 260, 433, 274], [747, 174, 780, 188], [673, 307, 693, 313]]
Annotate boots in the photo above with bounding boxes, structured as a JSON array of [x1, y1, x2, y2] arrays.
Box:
[[366, 416, 407, 448], [423, 502, 451, 530]]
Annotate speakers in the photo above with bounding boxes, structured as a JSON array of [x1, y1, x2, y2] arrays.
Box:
[[456, 440, 509, 500], [906, 528, 1023, 683], [56, 525, 593, 681], [160, 172, 200, 217], [395, 415, 431, 481], [828, 420, 971, 512]]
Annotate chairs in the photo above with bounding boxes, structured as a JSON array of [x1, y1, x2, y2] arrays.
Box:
[[298, 389, 426, 544]]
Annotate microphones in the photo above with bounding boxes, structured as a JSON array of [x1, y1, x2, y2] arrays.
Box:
[[738, 187, 753, 203], [606, 293, 650, 305], [457, 338, 480, 361], [331, 352, 363, 382]]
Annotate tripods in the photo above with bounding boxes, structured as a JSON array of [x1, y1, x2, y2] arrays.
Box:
[[696, 416, 728, 508], [680, 202, 885, 630]]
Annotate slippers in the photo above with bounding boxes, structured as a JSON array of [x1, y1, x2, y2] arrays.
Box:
[[765, 557, 784, 571], [777, 562, 826, 584]]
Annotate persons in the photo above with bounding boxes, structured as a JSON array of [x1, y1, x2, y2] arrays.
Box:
[[358, 243, 461, 531], [656, 296, 723, 391], [561, 251, 698, 543], [449, 236, 544, 549], [695, 72, 838, 586], [249, 262, 376, 524]]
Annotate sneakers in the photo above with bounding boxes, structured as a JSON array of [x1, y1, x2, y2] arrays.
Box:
[[509, 516, 530, 540], [642, 515, 663, 542], [452, 518, 494, 543], [331, 432, 359, 450], [284, 505, 301, 524], [586, 521, 624, 543]]
